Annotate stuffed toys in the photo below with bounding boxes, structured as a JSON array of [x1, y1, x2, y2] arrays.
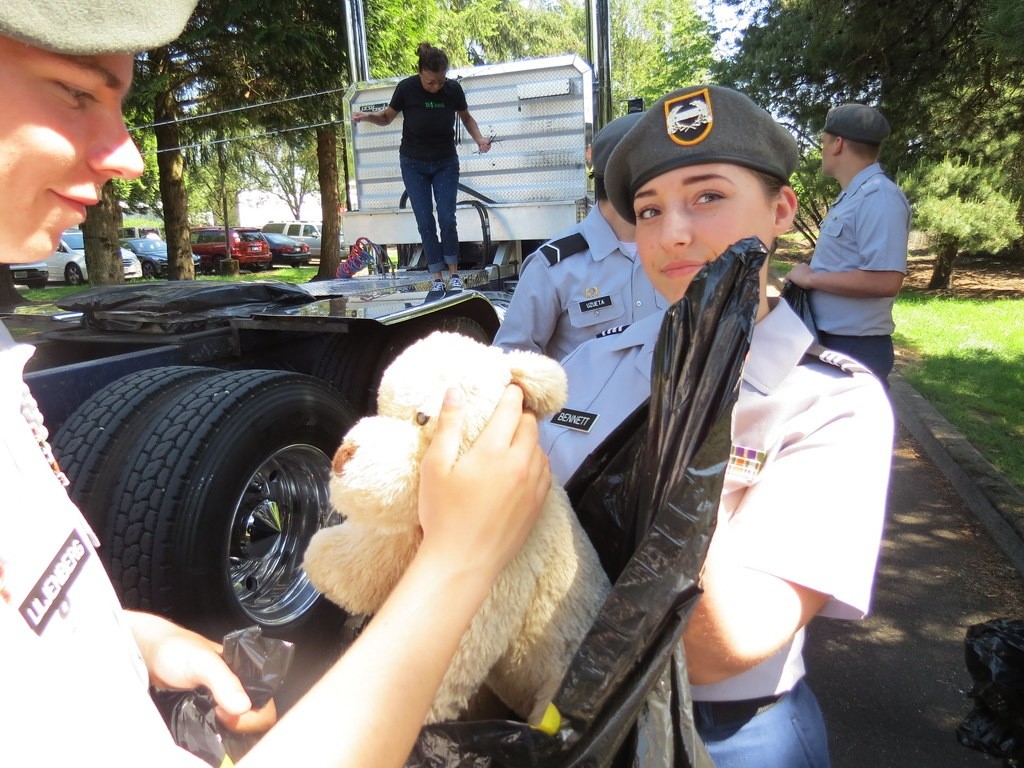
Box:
[[307, 325, 616, 727]]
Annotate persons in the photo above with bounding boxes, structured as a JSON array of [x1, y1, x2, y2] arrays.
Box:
[[350, 44, 496, 296], [534, 87, 895, 763], [0, 0, 552, 765], [779, 100, 908, 390], [493, 113, 678, 361]]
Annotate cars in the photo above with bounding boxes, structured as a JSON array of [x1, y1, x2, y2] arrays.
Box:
[[117, 236, 203, 280], [260, 232, 310, 268], [39, 226, 145, 283], [9, 262, 48, 289]]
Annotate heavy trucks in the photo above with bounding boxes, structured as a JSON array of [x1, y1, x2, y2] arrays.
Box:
[[11, 0, 619, 653]]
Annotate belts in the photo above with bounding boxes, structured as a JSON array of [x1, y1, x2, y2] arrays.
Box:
[[695, 696, 787, 730]]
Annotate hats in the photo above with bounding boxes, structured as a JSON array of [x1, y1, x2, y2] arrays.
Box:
[[604, 85, 799, 227], [592, 113, 648, 179], [0, 0, 199, 55], [824, 104, 890, 146]]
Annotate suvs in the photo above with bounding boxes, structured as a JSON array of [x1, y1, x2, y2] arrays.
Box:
[[260, 219, 352, 260], [191, 225, 275, 273], [119, 226, 169, 242]]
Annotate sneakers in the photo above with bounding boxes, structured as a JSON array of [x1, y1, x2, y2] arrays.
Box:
[[430, 275, 464, 290]]
